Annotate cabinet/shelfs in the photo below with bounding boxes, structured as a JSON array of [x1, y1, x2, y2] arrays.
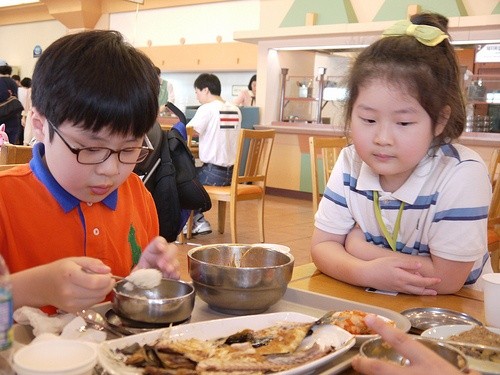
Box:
[[279, 69, 349, 125]]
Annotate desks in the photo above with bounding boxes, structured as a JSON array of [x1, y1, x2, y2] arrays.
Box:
[[177, 145, 199, 243]]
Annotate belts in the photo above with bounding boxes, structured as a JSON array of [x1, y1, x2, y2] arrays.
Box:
[[203, 162, 234, 171]]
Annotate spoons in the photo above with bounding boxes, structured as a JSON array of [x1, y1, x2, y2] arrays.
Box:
[[84, 266, 161, 290]]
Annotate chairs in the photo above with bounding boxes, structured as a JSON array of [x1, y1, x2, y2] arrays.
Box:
[[309, 137, 347, 213], [187, 128, 275, 245], [487, 149, 500, 273]]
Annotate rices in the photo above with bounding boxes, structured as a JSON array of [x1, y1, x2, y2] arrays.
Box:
[[123, 268, 162, 291]]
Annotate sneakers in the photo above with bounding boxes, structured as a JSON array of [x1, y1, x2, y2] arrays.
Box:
[[183, 213, 212, 236]]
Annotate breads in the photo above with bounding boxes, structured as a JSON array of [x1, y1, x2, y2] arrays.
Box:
[[445, 325, 500, 362]]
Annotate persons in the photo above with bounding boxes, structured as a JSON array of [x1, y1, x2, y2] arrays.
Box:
[[310, 12, 494, 296], [181, 73, 242, 239], [352, 313, 462, 375], [236, 74, 257, 107], [156, 68, 174, 117], [0, 66, 32, 146], [0, 30, 182, 324]]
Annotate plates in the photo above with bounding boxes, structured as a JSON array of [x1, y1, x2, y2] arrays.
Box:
[[420, 324, 500, 375], [103, 308, 191, 335], [399, 307, 483, 335], [96, 311, 356, 375]]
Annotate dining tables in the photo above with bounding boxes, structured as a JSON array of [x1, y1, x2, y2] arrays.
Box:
[[0, 262, 500, 375]]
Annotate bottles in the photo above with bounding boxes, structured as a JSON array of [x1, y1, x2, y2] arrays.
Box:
[[0, 255, 14, 374]]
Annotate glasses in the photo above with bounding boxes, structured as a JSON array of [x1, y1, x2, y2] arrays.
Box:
[[45, 114, 154, 165]]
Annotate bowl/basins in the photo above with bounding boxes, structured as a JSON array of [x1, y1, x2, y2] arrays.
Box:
[[186, 243, 296, 316], [111, 279, 196, 323], [321, 311, 398, 348], [360, 334, 469, 375]]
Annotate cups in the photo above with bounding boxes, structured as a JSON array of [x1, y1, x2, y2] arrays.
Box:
[[482, 273, 500, 329], [11, 338, 97, 375]]
